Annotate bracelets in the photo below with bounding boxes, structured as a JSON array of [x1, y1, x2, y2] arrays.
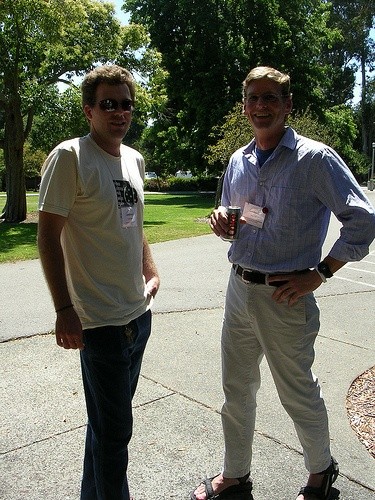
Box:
[[56, 304, 74, 312], [314, 265, 327, 282]]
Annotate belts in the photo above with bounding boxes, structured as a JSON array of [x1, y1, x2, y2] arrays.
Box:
[[233, 264, 286, 287]]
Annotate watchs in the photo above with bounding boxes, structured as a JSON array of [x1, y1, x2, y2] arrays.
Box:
[[318, 262, 333, 278]]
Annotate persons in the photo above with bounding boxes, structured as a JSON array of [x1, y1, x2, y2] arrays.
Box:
[[191, 66, 375, 500], [37, 66, 160, 500]]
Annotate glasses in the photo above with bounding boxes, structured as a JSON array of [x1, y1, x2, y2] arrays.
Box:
[[100, 98, 133, 112]]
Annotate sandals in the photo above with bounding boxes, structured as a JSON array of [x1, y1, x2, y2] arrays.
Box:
[[295, 456, 340, 500], [190, 471, 253, 500]]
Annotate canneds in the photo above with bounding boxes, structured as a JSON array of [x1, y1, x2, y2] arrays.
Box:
[[222, 206, 242, 241]]
